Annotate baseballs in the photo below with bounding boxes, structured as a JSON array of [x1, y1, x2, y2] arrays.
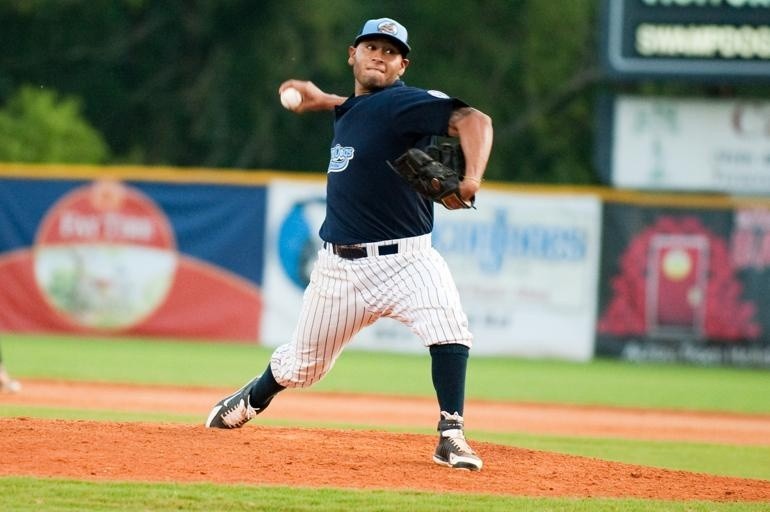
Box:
[[280, 86, 301, 110]]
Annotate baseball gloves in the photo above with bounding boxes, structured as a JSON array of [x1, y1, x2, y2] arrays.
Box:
[[385, 142, 477, 210]]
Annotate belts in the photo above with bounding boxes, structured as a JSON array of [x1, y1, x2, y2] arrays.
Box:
[[324, 241, 398, 258]]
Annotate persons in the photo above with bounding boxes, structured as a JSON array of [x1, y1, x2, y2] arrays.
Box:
[[206, 17, 495, 471]]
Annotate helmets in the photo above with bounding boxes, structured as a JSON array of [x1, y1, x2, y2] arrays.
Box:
[[353, 18, 411, 58]]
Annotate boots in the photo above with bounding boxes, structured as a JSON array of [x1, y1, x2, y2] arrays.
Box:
[[206, 376, 276, 429], [433, 411, 483, 472]]
